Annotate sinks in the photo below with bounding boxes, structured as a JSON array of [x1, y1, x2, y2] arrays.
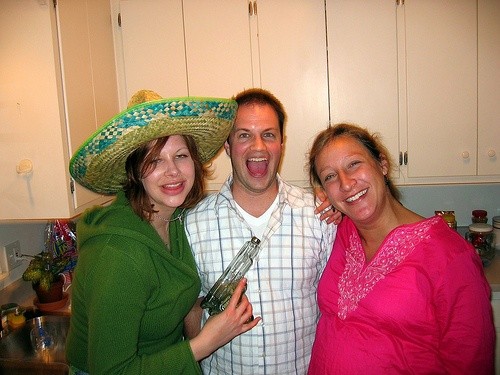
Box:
[[0, 359, 70, 375], [0, 309, 71, 369]]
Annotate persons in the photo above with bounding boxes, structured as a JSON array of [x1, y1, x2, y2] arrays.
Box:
[[183, 89, 340, 375], [65, 89, 342, 375], [305, 124, 497, 375]]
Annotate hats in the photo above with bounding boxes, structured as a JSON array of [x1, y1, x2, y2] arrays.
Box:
[[69, 89, 239, 198]]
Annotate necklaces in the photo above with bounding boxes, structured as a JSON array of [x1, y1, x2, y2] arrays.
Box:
[[165, 240, 168, 248]]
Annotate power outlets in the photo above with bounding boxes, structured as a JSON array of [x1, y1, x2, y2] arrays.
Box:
[[3, 240, 23, 271]]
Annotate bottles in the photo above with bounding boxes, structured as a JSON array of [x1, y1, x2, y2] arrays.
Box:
[[465, 222, 496, 268], [492, 216, 500, 250], [471, 210, 488, 225], [434, 210, 457, 232]]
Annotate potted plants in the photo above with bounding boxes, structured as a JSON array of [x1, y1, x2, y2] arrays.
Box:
[[23, 251, 71, 312]]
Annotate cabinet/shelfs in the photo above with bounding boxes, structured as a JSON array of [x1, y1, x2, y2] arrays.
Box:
[[0, 0, 500, 223]]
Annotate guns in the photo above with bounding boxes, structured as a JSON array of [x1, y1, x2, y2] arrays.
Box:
[[199, 237, 261, 323]]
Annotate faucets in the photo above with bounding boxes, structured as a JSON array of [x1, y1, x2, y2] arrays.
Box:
[[1, 306, 21, 336]]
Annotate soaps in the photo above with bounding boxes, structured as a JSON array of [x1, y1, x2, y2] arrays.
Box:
[[7, 313, 25, 325]]
